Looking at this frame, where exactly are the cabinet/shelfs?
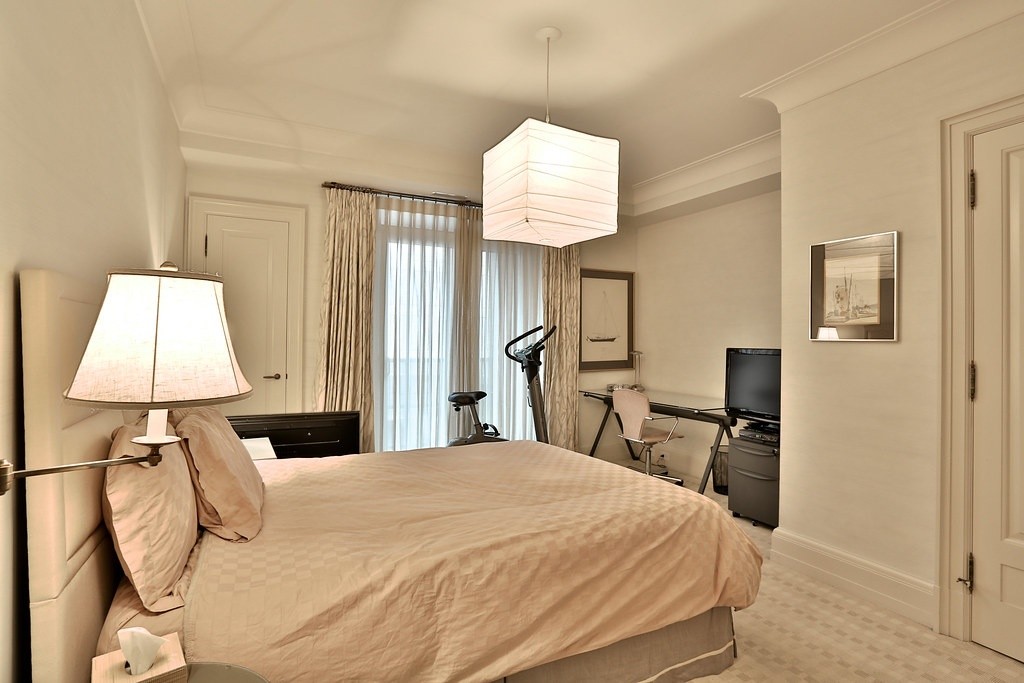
[729,438,780,530]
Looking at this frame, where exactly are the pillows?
[102,401,267,615]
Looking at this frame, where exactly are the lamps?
[1,262,254,495]
[483,26,620,249]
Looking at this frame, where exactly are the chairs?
[612,388,684,486]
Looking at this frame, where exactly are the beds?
[96,441,762,683]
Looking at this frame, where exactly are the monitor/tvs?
[725,348,780,434]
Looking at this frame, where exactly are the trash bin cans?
[710,444,730,496]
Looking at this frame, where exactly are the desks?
[579,387,738,495]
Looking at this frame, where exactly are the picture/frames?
[809,230,900,342]
[579,268,635,373]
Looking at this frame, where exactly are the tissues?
[91,627,188,683]
